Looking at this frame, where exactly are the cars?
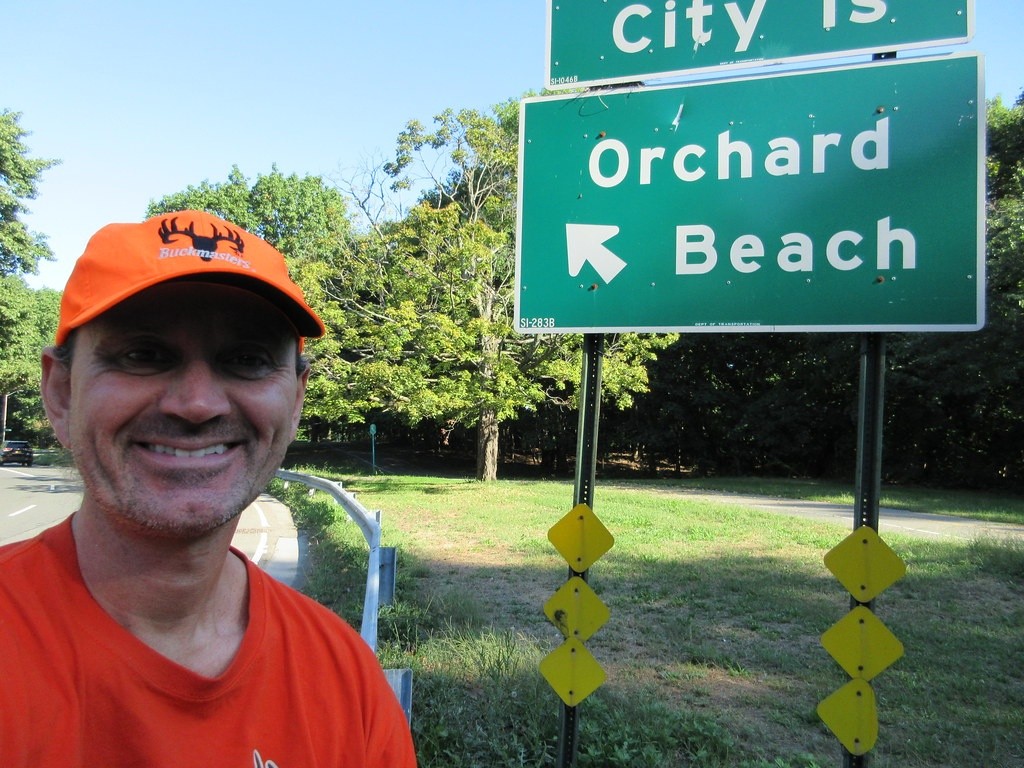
[0,441,33,467]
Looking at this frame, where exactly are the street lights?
[2,390,24,441]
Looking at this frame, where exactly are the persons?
[0,209,417,767]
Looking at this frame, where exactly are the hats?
[55,209,324,354]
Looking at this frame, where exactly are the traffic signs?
[512,0,987,335]
